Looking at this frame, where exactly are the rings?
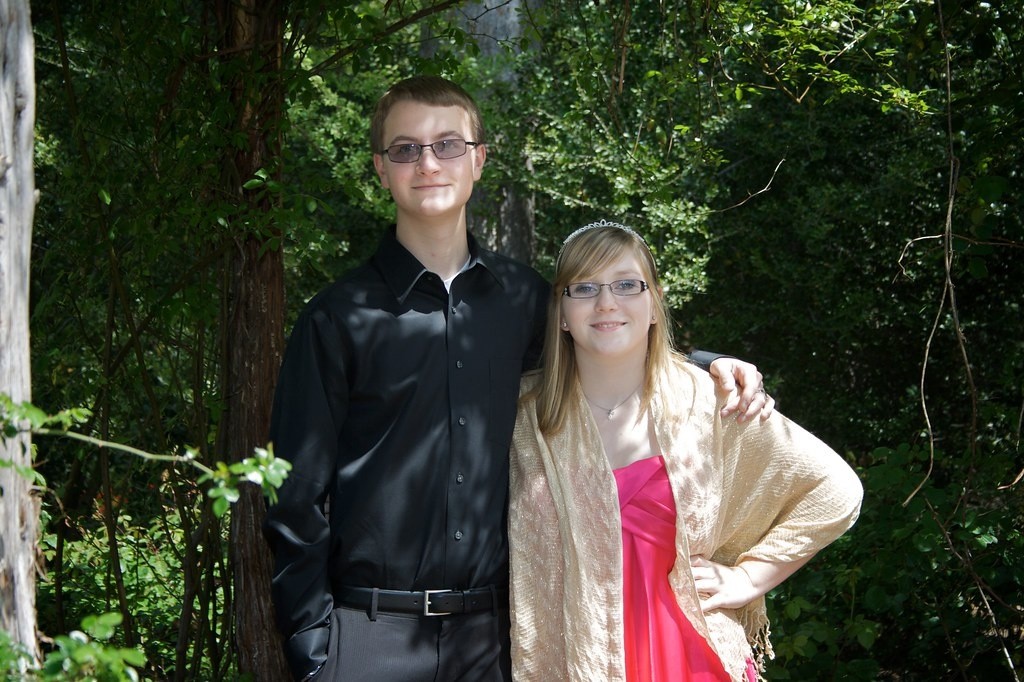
[756,390,768,400]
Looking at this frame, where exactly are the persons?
[261,76,775,682]
[507,218,863,682]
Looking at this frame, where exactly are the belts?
[338,583,509,617]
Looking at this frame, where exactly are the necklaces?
[584,377,644,421]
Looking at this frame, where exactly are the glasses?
[562,279,649,299]
[381,139,478,163]
